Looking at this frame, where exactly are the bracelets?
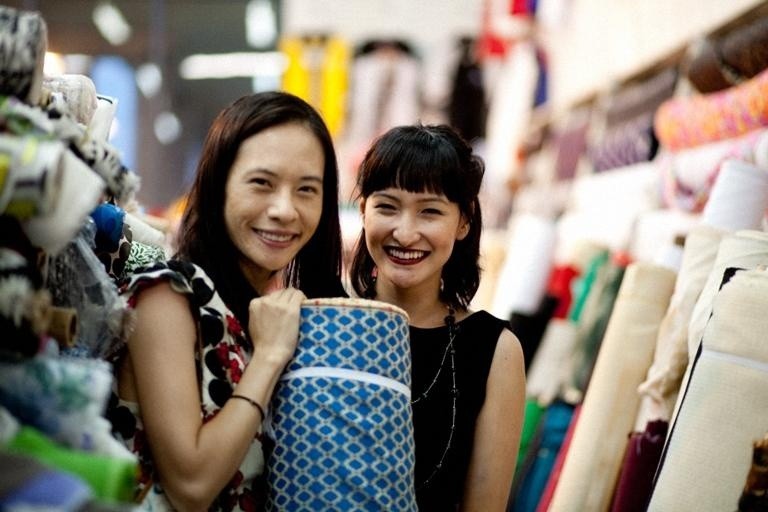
[223,390,273,424]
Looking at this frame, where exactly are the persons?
[344,121,529,512]
[94,85,348,509]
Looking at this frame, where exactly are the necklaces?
[402,303,466,495]
